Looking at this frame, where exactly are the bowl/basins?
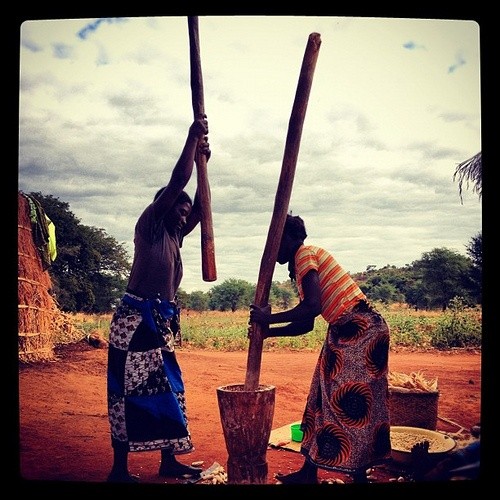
[290,423,306,442]
[387,425,457,464]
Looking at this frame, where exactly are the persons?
[247,213,391,493]
[105,112,212,485]
[409,441,481,494]
